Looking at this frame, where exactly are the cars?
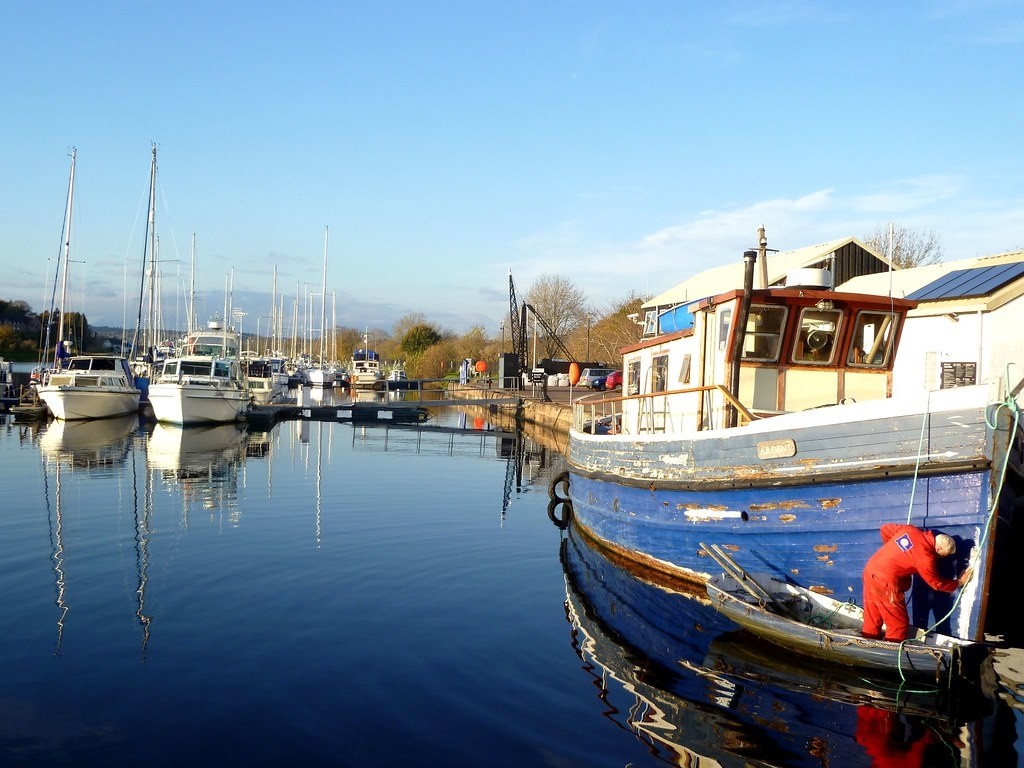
[591,375,607,392]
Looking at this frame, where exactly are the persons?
[859,523,971,644]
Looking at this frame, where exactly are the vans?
[579,367,615,390]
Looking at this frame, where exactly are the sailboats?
[1,143,407,423]
[21,423,337,662]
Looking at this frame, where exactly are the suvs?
[605,369,623,394]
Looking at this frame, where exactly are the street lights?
[500,325,505,352]
[586,312,591,366]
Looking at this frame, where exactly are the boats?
[549,502,1024,768]
[548,219,1023,687]
